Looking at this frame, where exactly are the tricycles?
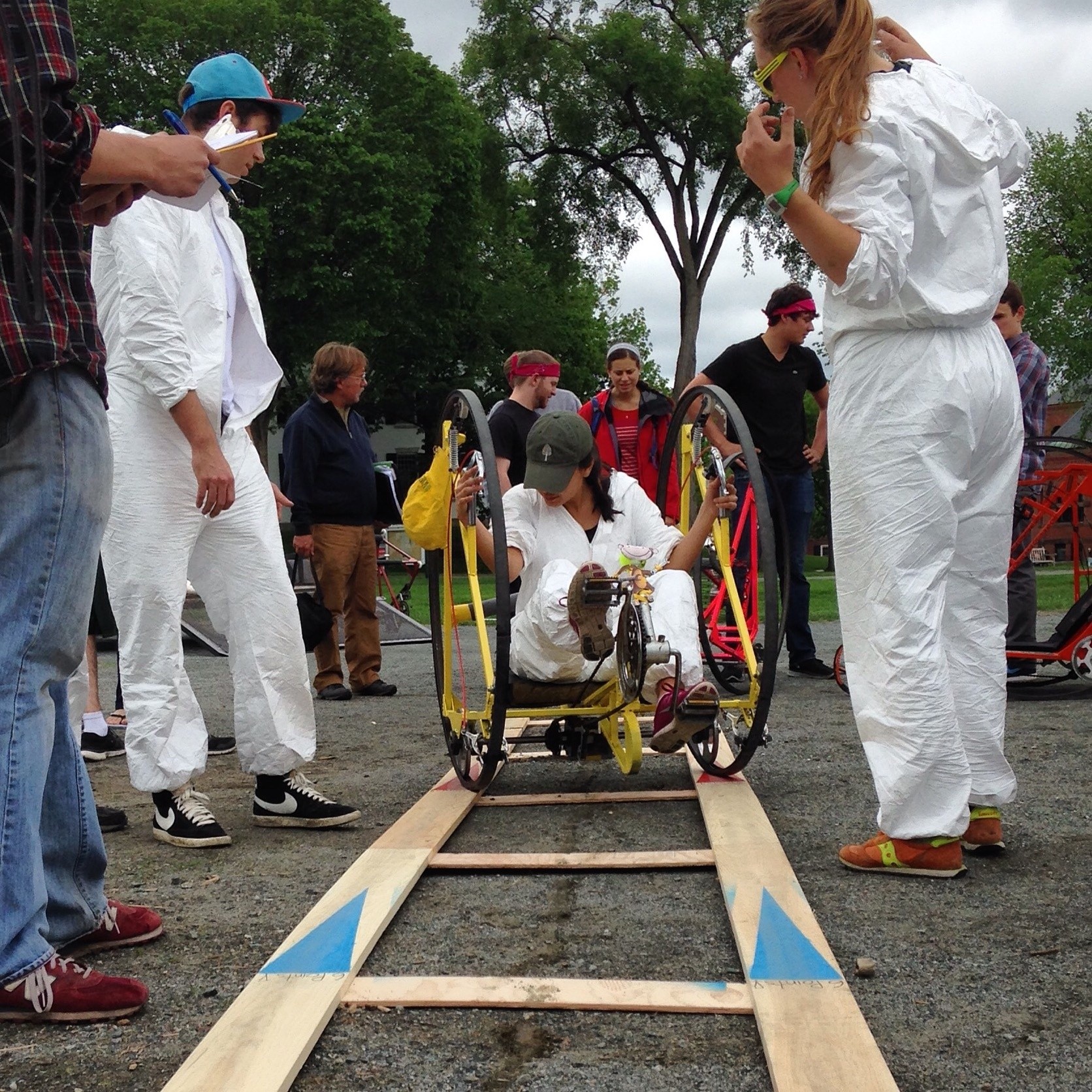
[834,432,1092,695]
[429,386,781,793]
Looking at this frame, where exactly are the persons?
[680,285,829,679]
[577,343,681,526]
[78,612,234,761]
[90,54,365,847]
[283,341,400,696]
[489,389,582,417]
[993,279,1050,674]
[455,409,737,754]
[736,0,1033,877]
[486,350,560,613]
[0,0,220,1025]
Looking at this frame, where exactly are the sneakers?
[1007,666,1037,680]
[0,956,149,1020]
[559,561,615,662]
[252,772,361,827]
[80,726,126,760]
[650,682,719,754]
[206,734,236,754]
[961,803,1006,853]
[95,804,128,831]
[722,667,745,682]
[839,830,966,878]
[56,899,161,960]
[787,657,834,679]
[355,679,397,696]
[153,789,232,847]
[317,683,351,700]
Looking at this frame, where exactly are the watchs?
[764,180,798,214]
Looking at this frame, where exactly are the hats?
[183,53,306,126]
[402,447,452,551]
[523,410,592,494]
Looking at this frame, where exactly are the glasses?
[344,373,367,383]
[754,49,788,96]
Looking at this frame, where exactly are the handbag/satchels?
[372,461,406,524]
[295,591,333,653]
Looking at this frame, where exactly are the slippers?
[109,712,126,726]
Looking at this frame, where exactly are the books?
[373,458,401,525]
